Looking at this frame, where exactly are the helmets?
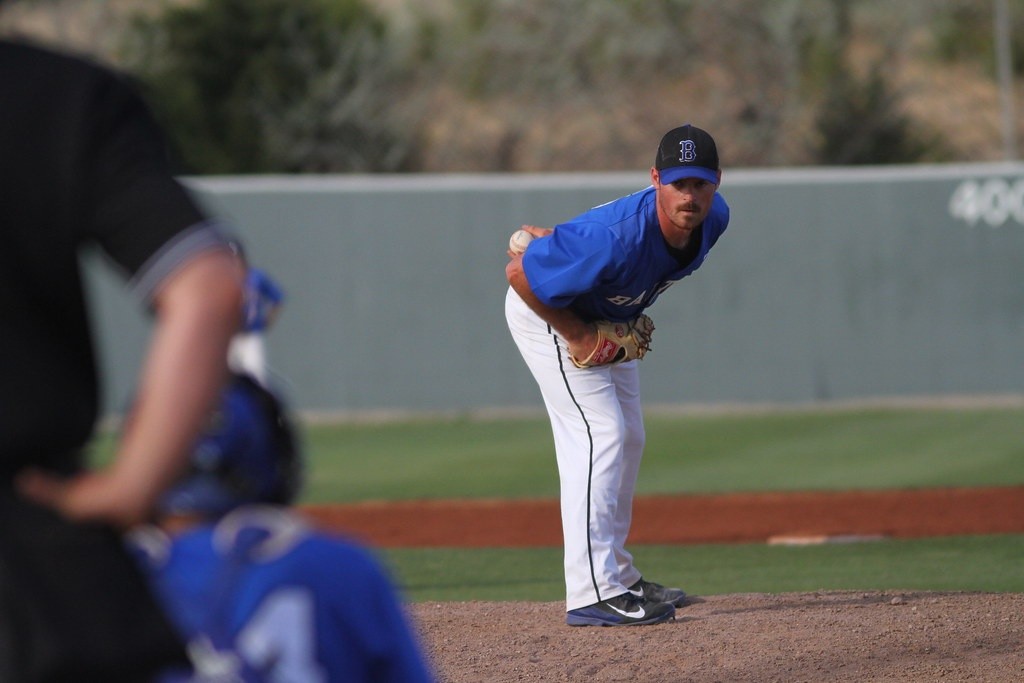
[155,369,303,520]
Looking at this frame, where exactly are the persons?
[0,38,247,683]
[226,237,284,382]
[125,375,435,683]
[504,123,729,626]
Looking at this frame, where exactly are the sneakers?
[566,591,675,626]
[627,578,685,604]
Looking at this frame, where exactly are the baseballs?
[510,229,534,254]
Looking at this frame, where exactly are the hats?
[656,122,720,186]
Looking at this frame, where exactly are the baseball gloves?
[566,313,655,369]
